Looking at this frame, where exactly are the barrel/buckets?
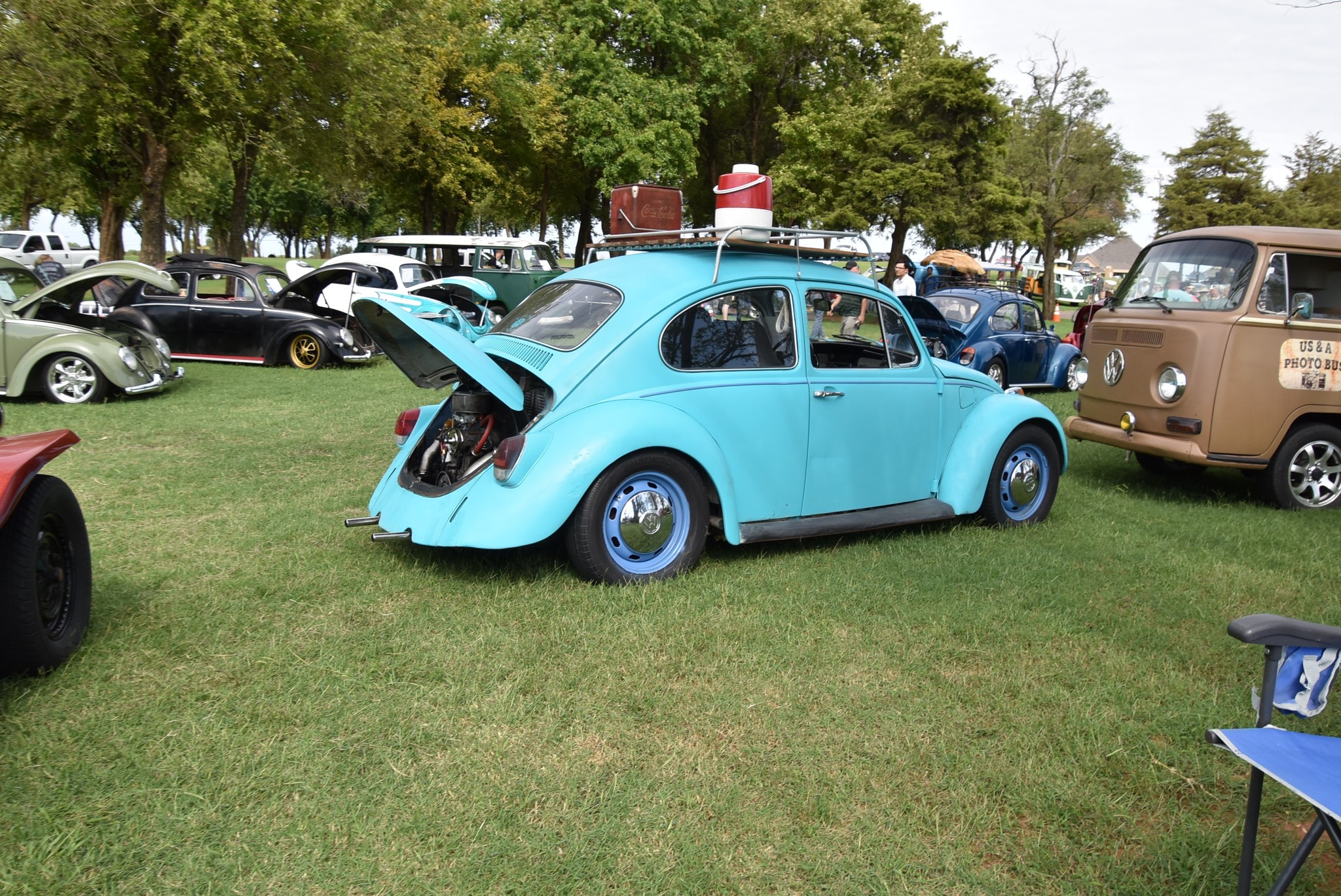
[712,164,774,243]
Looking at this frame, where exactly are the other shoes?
[819,336,829,342]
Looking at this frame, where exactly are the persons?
[1091,272,1105,303]
[892,260,917,298]
[488,249,506,269]
[808,260,833,341]
[33,254,67,293]
[995,271,1009,291]
[961,271,978,289]
[919,267,939,296]
[1129,269,1232,303]
[826,261,868,349]
[720,295,732,321]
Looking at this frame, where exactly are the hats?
[1139,278,1151,283]
[1092,275,1097,279]
[843,261,858,269]
[1096,272,1101,275]
[1196,286,1211,293]
[1166,271,1182,280]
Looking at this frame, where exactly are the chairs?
[711,319,783,367]
[675,308,713,329]
[227,297,247,301]
[1197,612,1341,896]
[207,297,226,300]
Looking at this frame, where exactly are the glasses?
[894,266,907,271]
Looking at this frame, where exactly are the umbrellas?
[919,249,986,287]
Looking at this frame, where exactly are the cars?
[0,230,187,403]
[1018,264,1091,303]
[346,228,1066,584]
[107,253,386,370]
[286,228,569,342]
[1062,225,1341,511]
[876,276,1082,393]
[1,405,93,678]
